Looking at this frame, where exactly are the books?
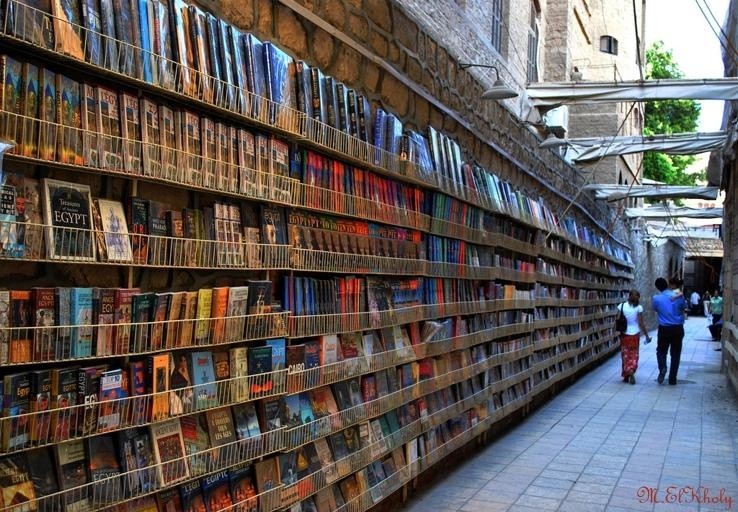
[1,1,633,512]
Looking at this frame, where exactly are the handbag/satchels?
[616,311,627,332]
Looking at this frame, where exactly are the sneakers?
[630,377,635,385]
[623,378,629,383]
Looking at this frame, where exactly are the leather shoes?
[658,368,667,384]
[669,380,677,385]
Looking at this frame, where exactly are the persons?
[667,277,683,301]
[615,289,649,386]
[651,277,683,385]
[685,285,723,342]
